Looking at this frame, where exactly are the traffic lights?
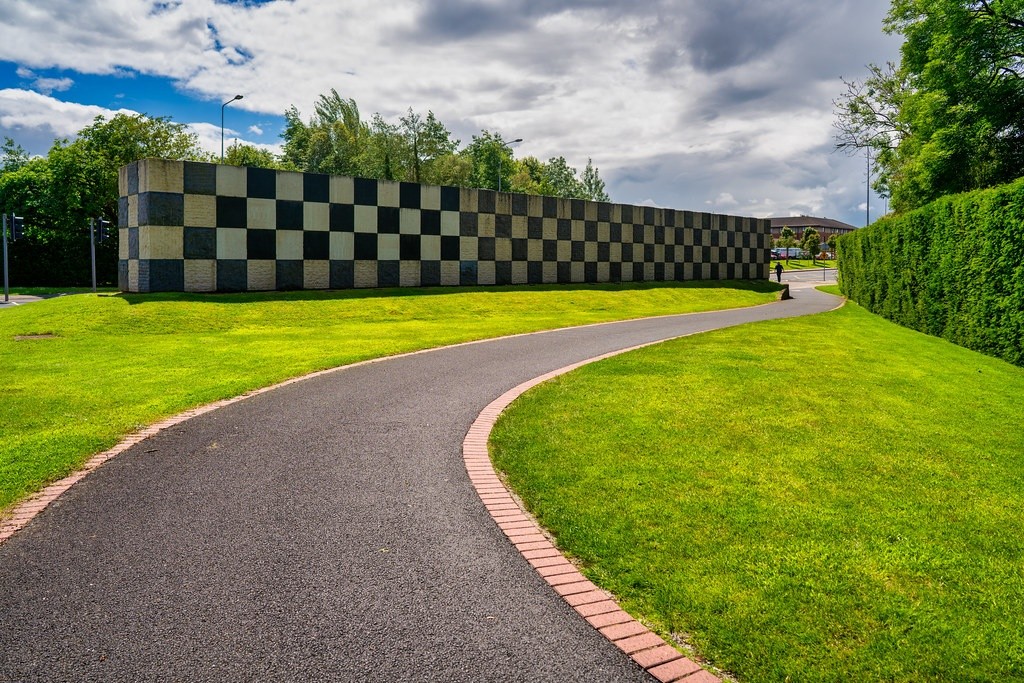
[97,216,109,244]
[11,212,25,242]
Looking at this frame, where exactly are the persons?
[775,262,784,282]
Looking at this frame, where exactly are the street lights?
[498,138,523,191]
[836,143,870,227]
[221,94,254,164]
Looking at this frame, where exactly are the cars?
[769,247,835,261]
